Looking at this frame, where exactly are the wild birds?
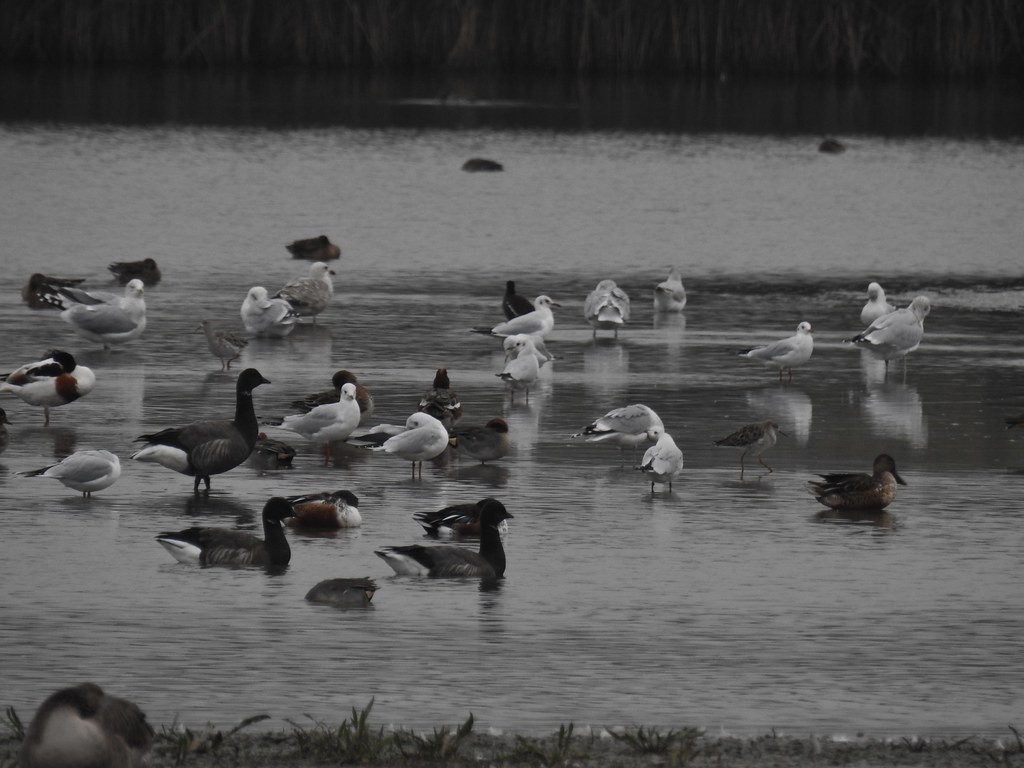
[16,450,122,500]
[9,682,160,768]
[0,348,97,427]
[155,496,303,571]
[19,258,162,311]
[193,137,932,613]
[130,368,272,502]
[59,279,148,356]
